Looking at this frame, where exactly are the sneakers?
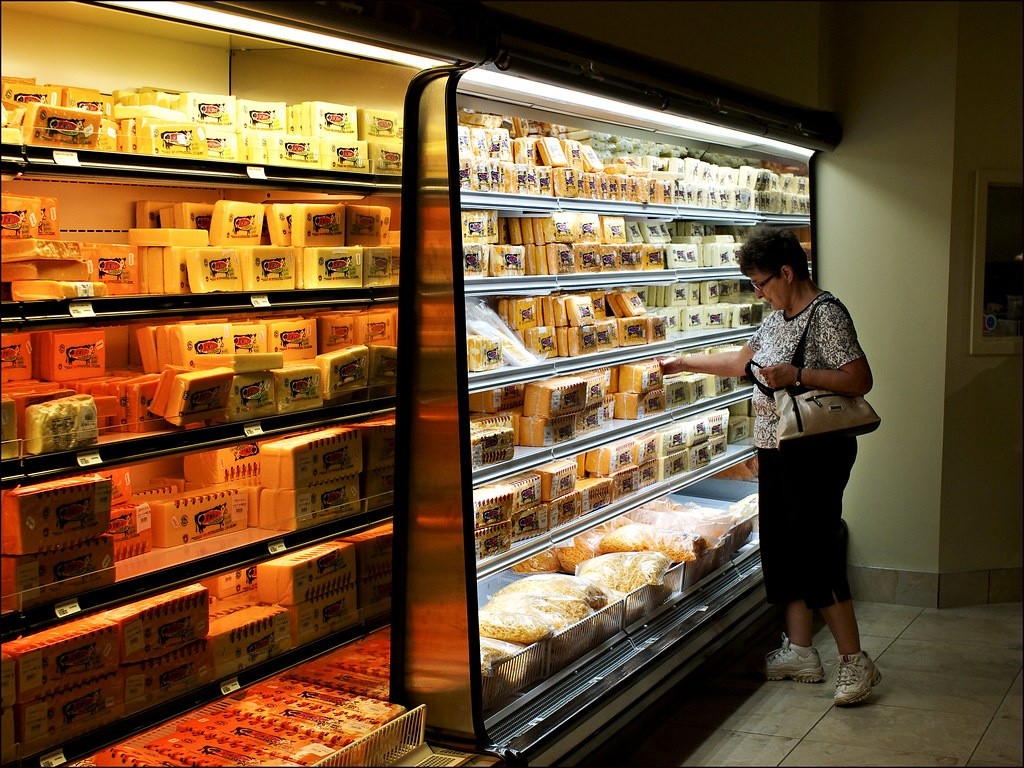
[753,631,824,683]
[824,650,881,704]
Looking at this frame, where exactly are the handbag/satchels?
[745,299,880,449]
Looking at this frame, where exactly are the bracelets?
[796,367,802,386]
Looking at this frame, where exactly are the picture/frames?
[969,170,1024,355]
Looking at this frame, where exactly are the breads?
[0,72,401,767]
[458,108,762,169]
[455,127,809,557]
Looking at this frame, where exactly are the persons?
[658,227,881,705]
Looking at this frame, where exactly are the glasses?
[750,271,779,291]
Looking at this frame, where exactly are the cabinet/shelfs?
[459,20,844,734]
[0,0,457,768]
